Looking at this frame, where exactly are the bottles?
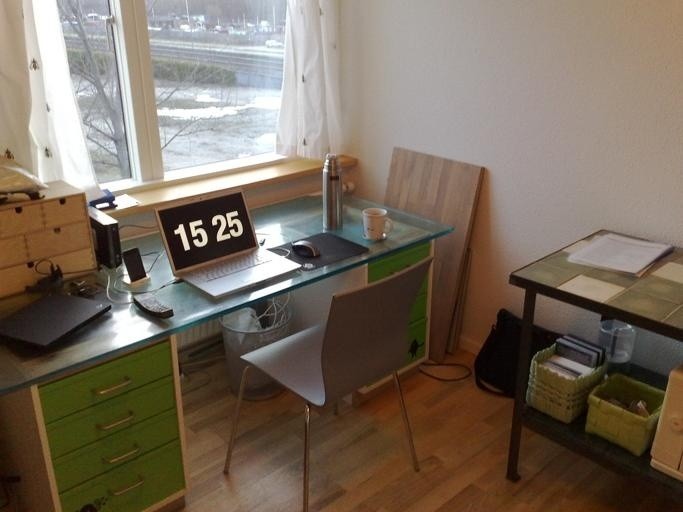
[321,152,344,230]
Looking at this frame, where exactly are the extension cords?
[33,275,63,292]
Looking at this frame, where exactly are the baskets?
[525,342,609,424]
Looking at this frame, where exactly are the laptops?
[0,291,115,349]
[155,187,302,299]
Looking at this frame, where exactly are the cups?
[360,207,393,241]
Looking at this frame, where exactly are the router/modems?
[87,206,122,268]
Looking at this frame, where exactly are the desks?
[0,190,453,511]
[506,228,682,502]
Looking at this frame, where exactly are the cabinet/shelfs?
[1,180,97,299]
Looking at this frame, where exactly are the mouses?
[293,240,320,257]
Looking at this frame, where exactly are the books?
[566,232,674,278]
[537,334,605,380]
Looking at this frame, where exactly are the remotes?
[133,292,174,319]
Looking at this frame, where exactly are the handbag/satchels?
[474,309,564,401]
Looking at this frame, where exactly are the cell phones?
[123,248,146,282]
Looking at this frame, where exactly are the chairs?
[220,254,433,512]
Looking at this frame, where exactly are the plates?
[361,232,386,240]
